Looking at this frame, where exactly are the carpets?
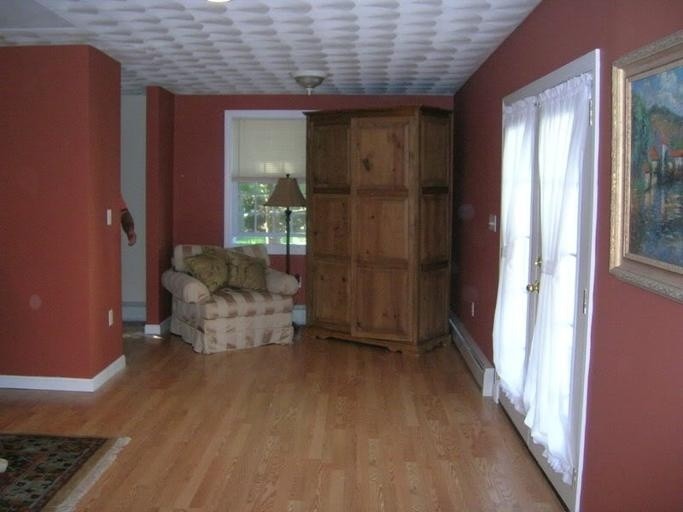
[0,433,133,511]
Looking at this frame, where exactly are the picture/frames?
[608,28,682,305]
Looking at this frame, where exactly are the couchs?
[161,241,300,358]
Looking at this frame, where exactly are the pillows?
[226,247,266,292]
[186,253,225,293]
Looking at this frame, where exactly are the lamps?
[264,174,305,275]
[288,68,327,96]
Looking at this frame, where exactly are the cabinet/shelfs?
[301,105,456,358]
